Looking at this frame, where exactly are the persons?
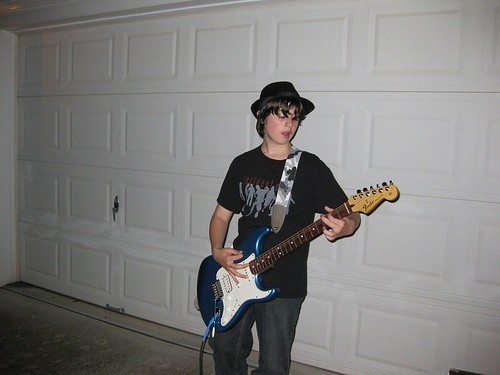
[209,81,361,375]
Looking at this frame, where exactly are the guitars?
[195,180,399,332]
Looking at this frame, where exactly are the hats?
[250,81,315,119]
